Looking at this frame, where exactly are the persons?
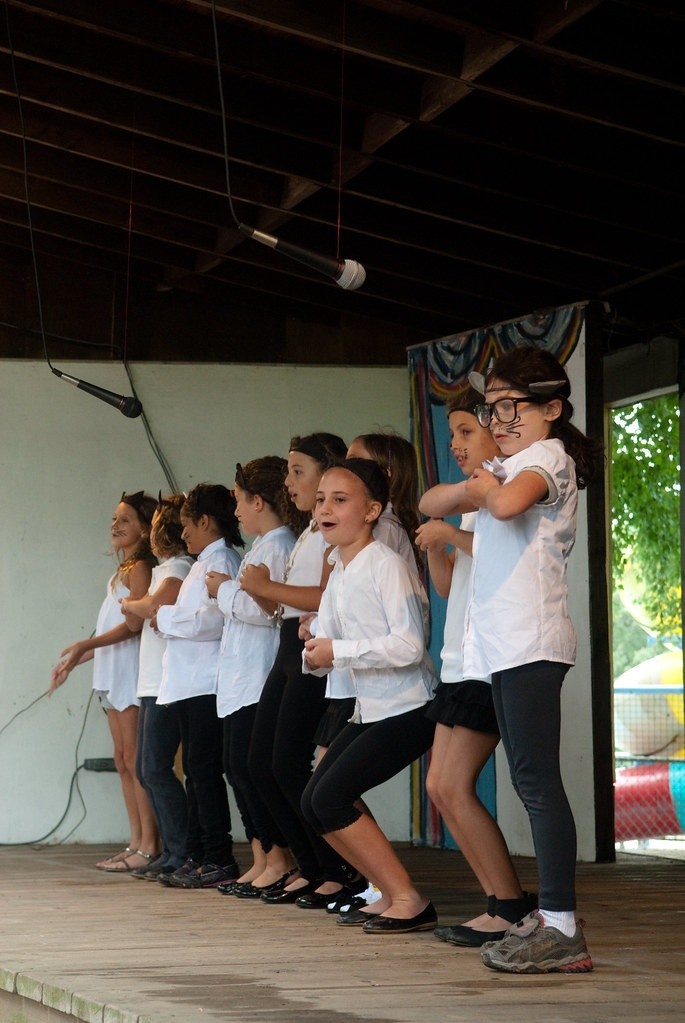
[261,432,348,908]
[418,345,608,974]
[204,456,311,898]
[149,483,246,888]
[414,391,538,947]
[47,488,197,881]
[300,457,439,933]
[298,433,426,916]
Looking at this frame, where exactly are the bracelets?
[262,601,284,621]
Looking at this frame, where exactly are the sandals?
[104,849,165,872]
[96,845,137,870]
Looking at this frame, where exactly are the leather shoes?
[259,874,321,904]
[233,865,301,897]
[218,873,250,894]
[295,876,369,908]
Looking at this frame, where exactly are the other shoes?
[362,901,438,932]
[433,910,496,942]
[336,908,378,927]
[170,862,242,888]
[447,919,508,948]
[157,858,203,886]
[130,854,162,878]
[143,855,181,881]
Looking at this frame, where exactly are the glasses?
[474,395,535,428]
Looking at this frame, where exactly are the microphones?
[236,222,366,292]
[51,369,142,419]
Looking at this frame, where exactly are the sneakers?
[480,909,593,973]
